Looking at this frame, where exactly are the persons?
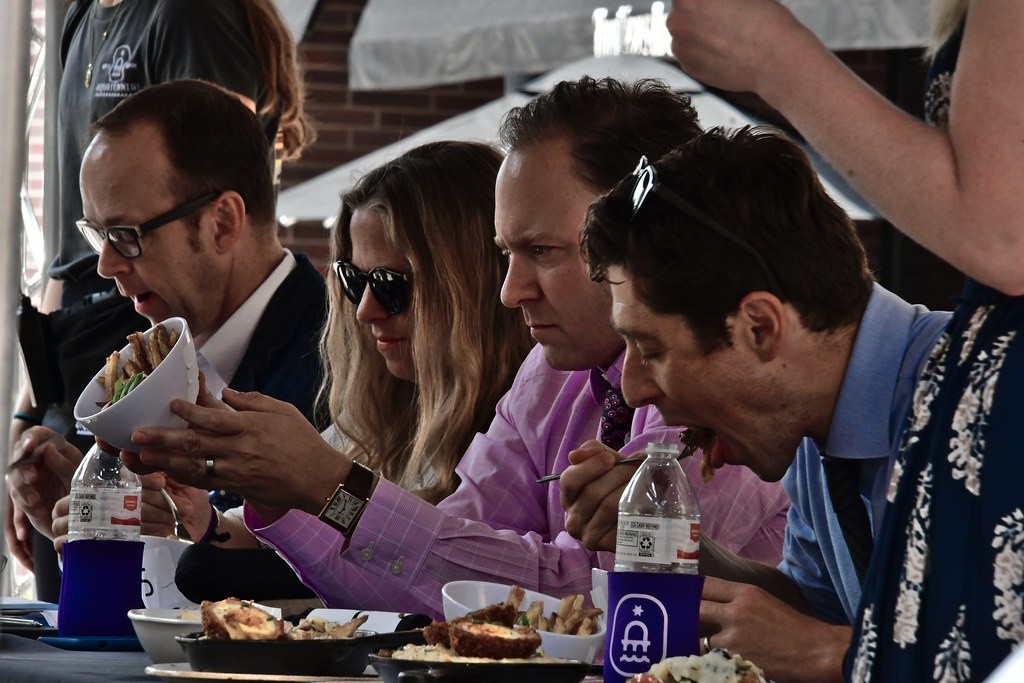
[666,1,1024,683]
[7,1,334,592]
[556,126,955,683]
[51,140,540,589]
[94,69,791,626]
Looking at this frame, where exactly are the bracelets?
[203,505,221,543]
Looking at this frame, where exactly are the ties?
[600,384,635,451]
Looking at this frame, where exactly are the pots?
[366,651,603,682]
[174,631,425,675]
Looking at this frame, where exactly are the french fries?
[97,324,179,411]
[505,586,603,635]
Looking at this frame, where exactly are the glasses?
[627,154,786,302]
[75,191,248,257]
[332,257,413,316]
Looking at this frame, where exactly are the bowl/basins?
[592,569,608,627]
[443,579,607,664]
[128,600,281,661]
[57,533,194,610]
[72,316,199,451]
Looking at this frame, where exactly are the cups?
[603,572,705,683]
[58,540,147,636]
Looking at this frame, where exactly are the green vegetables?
[112,371,146,404]
[396,601,542,657]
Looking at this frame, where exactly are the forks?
[536,430,705,486]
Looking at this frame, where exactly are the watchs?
[317,460,374,536]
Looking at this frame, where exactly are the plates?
[146,662,381,683]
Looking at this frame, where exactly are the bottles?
[69,440,142,541]
[614,441,700,575]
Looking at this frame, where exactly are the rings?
[204,458,214,477]
[703,635,711,653]
